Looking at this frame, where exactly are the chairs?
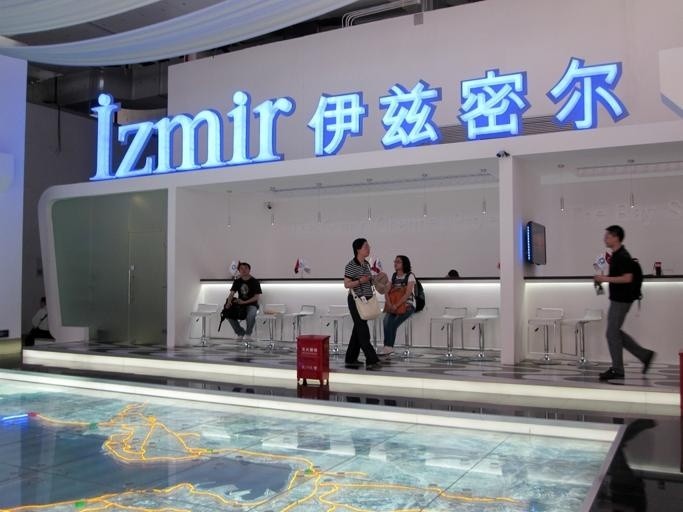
[256,302,287,352]
[463,307,500,361]
[431,305,468,361]
[371,306,424,359]
[235,303,262,349]
[283,304,316,353]
[319,304,350,356]
[190,302,219,348]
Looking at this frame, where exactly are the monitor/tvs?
[525,220,547,265]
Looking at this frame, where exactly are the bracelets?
[358,278,361,285]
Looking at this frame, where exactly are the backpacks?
[405,272,425,313]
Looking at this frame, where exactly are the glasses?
[393,260,403,264]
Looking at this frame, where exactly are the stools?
[527,308,603,366]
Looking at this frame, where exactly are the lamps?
[227,191,231,227]
[268,168,492,227]
[627,160,636,208]
[557,164,566,211]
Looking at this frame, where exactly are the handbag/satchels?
[630,257,642,299]
[384,287,407,313]
[223,298,248,320]
[354,295,381,320]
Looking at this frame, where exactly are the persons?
[25,295,52,346]
[225,264,263,342]
[592,225,655,380]
[343,238,388,368]
[376,255,416,358]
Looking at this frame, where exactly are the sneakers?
[236,334,252,342]
[599,367,623,379]
[641,350,653,374]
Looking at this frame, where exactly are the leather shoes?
[345,345,393,366]
